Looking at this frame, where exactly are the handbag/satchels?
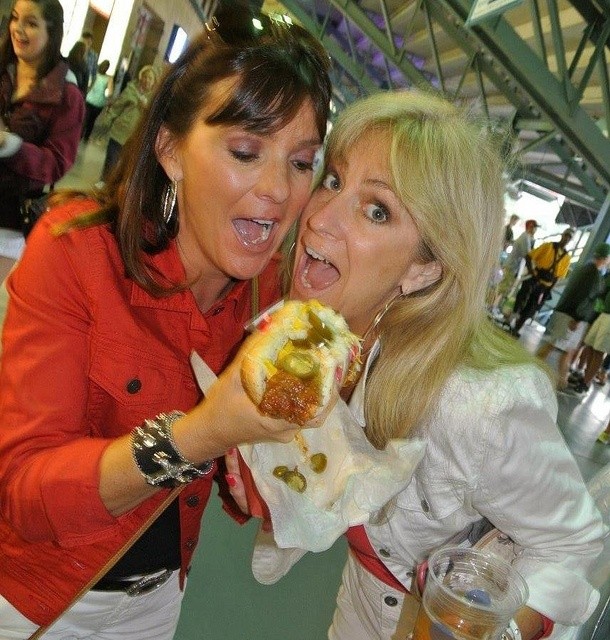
[16,183,54,243]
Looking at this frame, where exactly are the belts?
[90,570,176,599]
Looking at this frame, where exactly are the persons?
[80,57,110,144]
[493,220,541,315]
[287,83,609,639]
[65,42,89,96]
[0,0,88,233]
[534,241,610,399]
[505,227,576,340]
[80,31,98,77]
[1,19,335,636]
[502,214,523,250]
[568,300,610,394]
[93,60,160,188]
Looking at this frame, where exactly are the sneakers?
[596,432,609,444]
[556,383,576,398]
[567,372,589,396]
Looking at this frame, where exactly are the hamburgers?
[241,301,366,429]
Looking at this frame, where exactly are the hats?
[593,242,610,257]
[563,229,574,238]
[525,220,541,229]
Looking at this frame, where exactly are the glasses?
[185,7,331,74]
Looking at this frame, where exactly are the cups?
[412,546,530,640]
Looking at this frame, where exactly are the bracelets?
[128,408,217,493]
